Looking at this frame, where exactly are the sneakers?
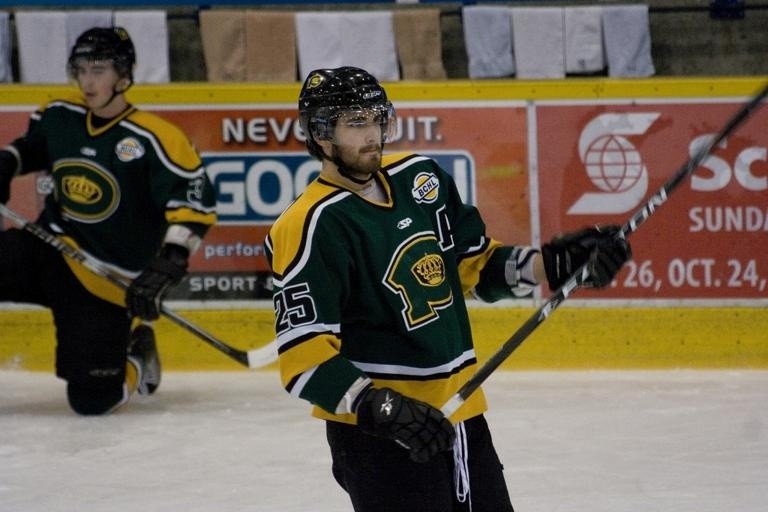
[132,324,160,396]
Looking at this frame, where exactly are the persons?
[263,67,632,512]
[0,27,217,414]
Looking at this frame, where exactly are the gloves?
[540,226,632,293]
[124,259,186,322]
[358,386,456,464]
[1,150,18,206]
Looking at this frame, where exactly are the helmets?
[298,66,388,139]
[68,27,136,65]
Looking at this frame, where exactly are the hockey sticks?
[0,205,278,370]
